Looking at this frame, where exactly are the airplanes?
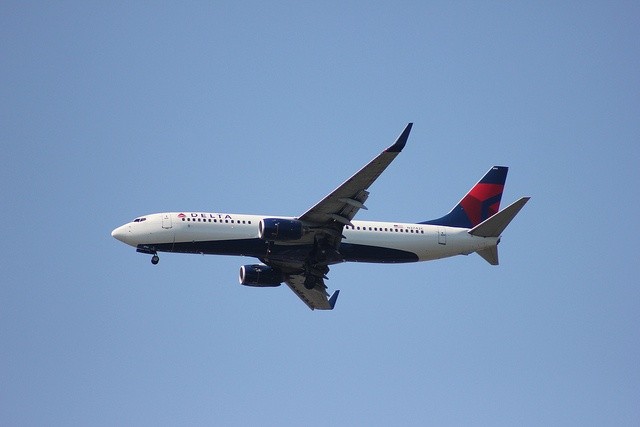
[110,122,530,311]
